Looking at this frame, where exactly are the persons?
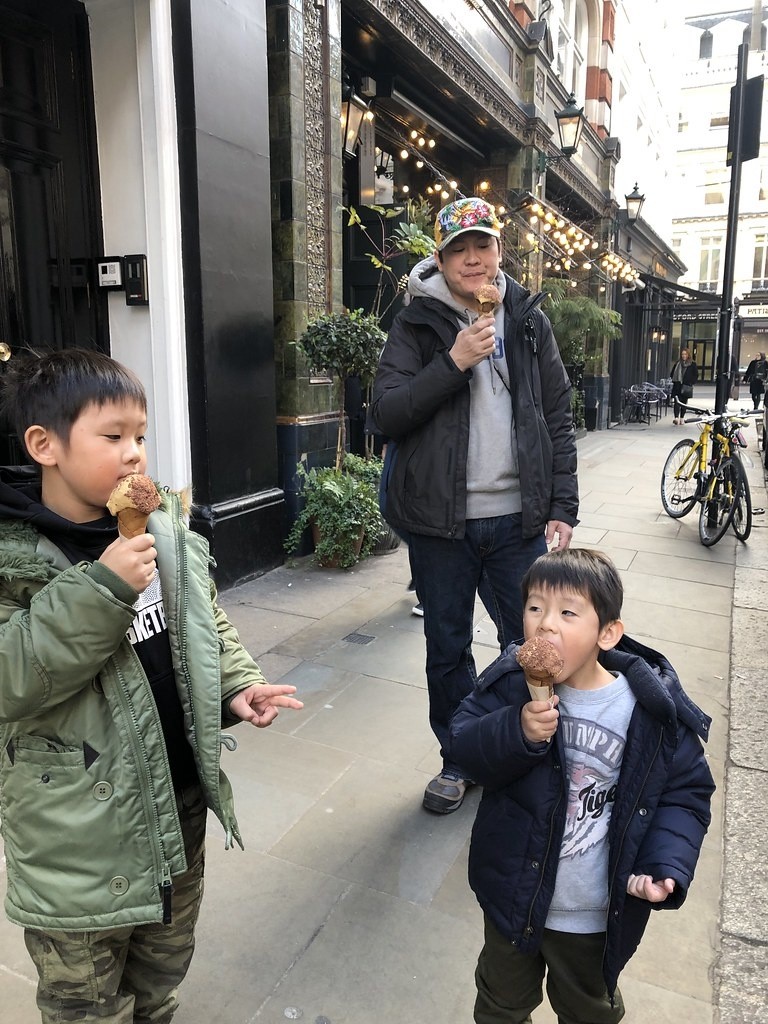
[0,353,307,1024]
[742,351,768,410]
[371,198,587,817]
[667,349,699,425]
[714,346,738,405]
[450,545,713,1024]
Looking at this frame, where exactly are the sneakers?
[423,771,476,815]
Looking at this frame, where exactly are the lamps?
[659,329,667,344]
[653,325,661,343]
[554,91,585,155]
[341,81,369,167]
[625,182,646,222]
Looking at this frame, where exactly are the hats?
[434,196,500,250]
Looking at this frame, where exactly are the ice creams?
[473,284,502,316]
[106,473,163,542]
[515,636,565,743]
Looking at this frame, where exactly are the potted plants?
[285,466,372,568]
[342,453,401,556]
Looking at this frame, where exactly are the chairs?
[631,385,657,425]
[642,381,667,419]
[658,378,674,408]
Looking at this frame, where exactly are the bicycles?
[673,408,765,541]
[660,396,751,548]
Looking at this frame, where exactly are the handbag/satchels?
[680,385,694,398]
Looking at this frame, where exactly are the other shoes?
[673,419,684,425]
